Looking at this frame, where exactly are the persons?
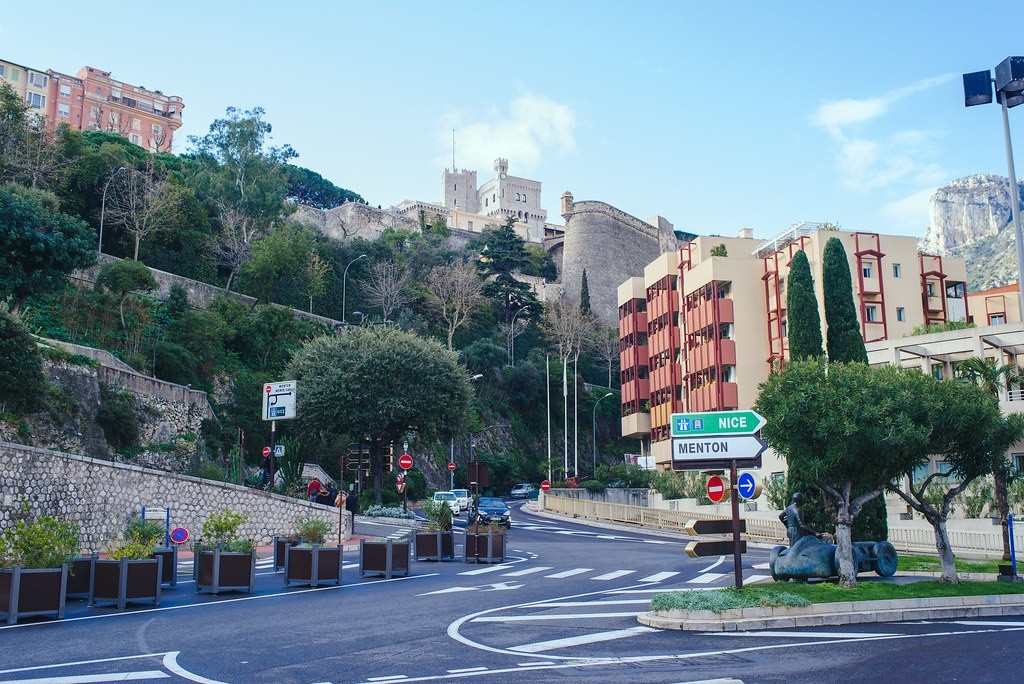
[777,492,822,547]
[334,490,347,510]
[346,492,358,516]
[307,477,321,502]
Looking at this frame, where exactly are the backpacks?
[311,483,318,496]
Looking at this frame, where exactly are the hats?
[313,477,317,480]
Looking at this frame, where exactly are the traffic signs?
[382,440,393,472]
[346,443,371,471]
[671,435,769,460]
[671,409,767,438]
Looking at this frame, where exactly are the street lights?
[451,373,483,489]
[593,392,613,480]
[98,167,126,254]
[353,311,364,329]
[343,255,368,322]
[512,305,532,368]
[470,424,511,461]
[962,55,1024,306]
[403,441,408,513]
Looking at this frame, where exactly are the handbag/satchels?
[336,495,346,506]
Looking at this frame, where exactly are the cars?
[509,484,535,498]
[449,489,474,511]
[467,497,512,529]
[432,491,461,516]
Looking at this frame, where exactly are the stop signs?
[707,476,730,503]
[448,462,455,470]
[541,480,550,491]
[266,386,271,392]
[399,455,414,470]
[262,446,271,457]
